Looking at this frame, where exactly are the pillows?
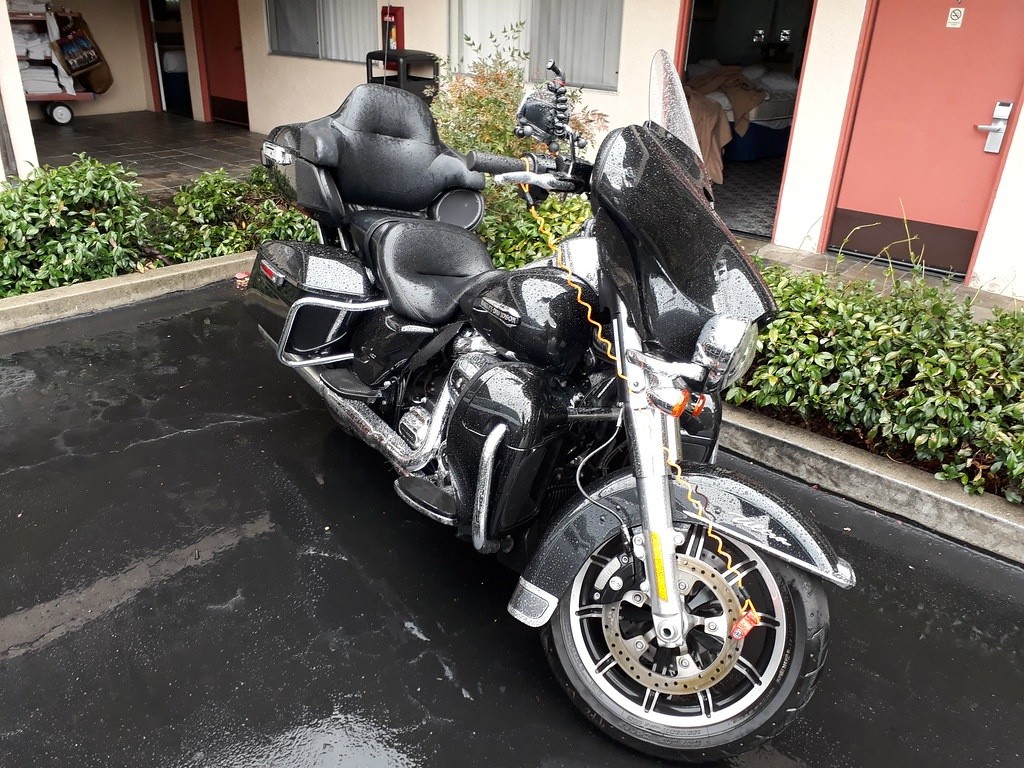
[761,71,798,91]
[742,63,769,80]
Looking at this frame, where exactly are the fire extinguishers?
[389,25,396,50]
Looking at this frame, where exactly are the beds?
[682,60,799,129]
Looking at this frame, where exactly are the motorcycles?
[239,48,861,768]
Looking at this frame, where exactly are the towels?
[6,0,77,96]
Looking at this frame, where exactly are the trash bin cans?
[366,49,440,107]
